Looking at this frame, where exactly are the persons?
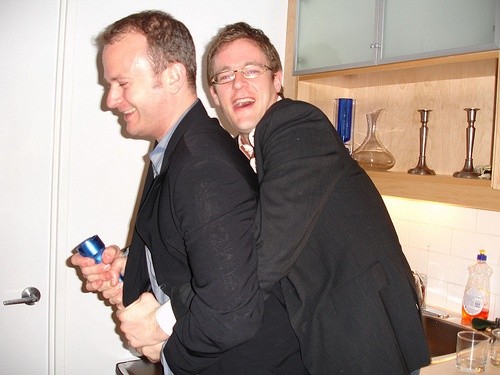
[71,9,307,375]
[97,22,432,375]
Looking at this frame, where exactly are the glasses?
[209,64,274,86]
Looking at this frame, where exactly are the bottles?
[350,108,395,170]
[462,250,492,325]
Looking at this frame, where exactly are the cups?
[490,328,500,365]
[456,331,490,373]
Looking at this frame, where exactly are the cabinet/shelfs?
[283,0,500,357]
